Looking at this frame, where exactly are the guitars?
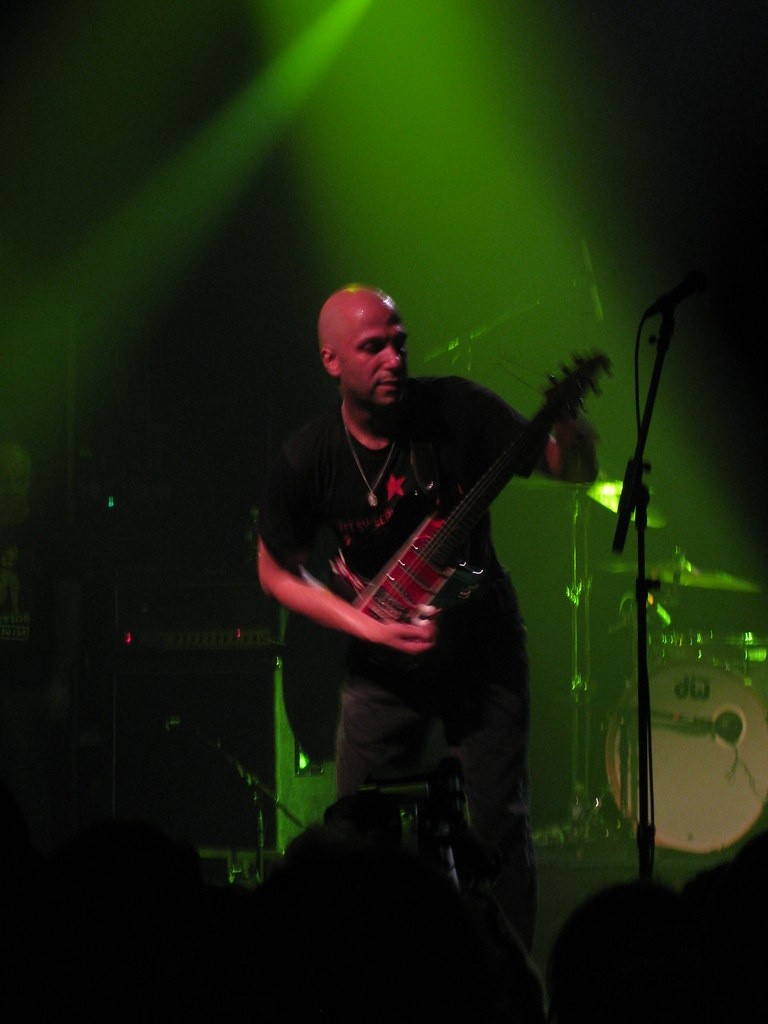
[322,345,616,672]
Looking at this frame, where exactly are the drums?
[597,651,768,856]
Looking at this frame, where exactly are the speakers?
[111,657,277,886]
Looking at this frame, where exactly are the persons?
[256,282,599,951]
[0,441,84,853]
[0,782,768,1024]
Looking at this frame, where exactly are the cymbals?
[585,479,671,529]
[589,546,764,596]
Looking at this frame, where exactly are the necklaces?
[345,411,397,506]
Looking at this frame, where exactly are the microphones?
[643,269,702,322]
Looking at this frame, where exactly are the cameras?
[355,776,471,856]
